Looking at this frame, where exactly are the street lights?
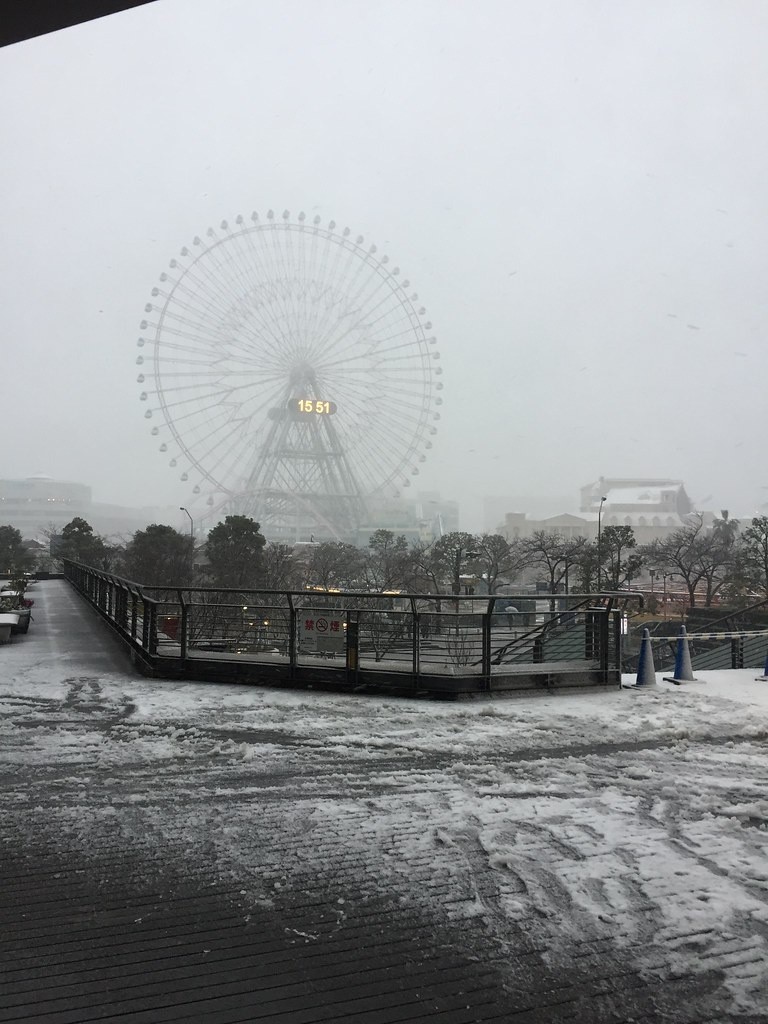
[455,552,482,637]
[663,575,666,621]
[181,507,193,539]
[650,569,654,595]
[598,497,607,591]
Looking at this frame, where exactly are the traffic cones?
[662,624,705,686]
[623,627,657,691]
